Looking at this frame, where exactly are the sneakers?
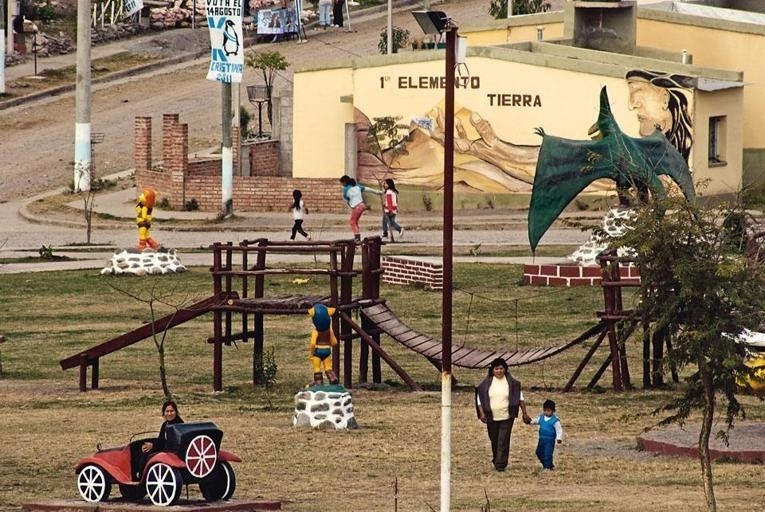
[399,228,404,237]
[381,236,387,241]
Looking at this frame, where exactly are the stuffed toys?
[308,302,339,385]
[134,188,162,252]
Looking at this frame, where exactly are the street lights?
[413,3,468,511]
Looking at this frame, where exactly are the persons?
[381,177,403,239]
[474,357,531,473]
[135,402,185,478]
[526,399,562,470]
[331,0,345,28]
[317,0,332,29]
[288,190,312,239]
[427,69,693,199]
[269,13,281,29]
[338,174,386,243]
